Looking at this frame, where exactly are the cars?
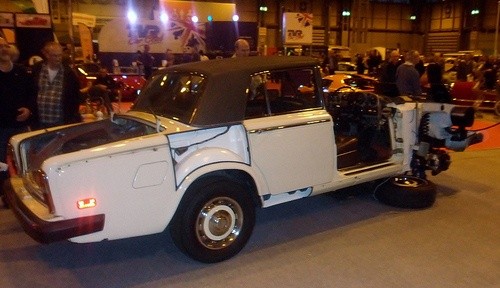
[296,73,380,96]
[321,62,361,74]
[448,78,500,113]
[424,58,467,73]
[7,56,484,263]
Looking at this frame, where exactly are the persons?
[64,34,500,104]
[4,44,34,100]
[92,67,117,119]
[0,37,36,209]
[28,41,82,137]
